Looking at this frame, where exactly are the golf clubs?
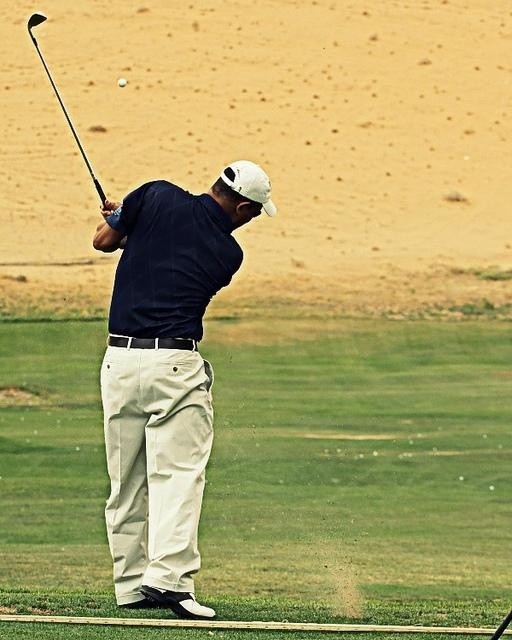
[29,14,106,204]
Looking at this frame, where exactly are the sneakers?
[141,585,215,620]
[118,595,170,610]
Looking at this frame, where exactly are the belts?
[107,331,198,352]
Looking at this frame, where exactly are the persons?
[91,158,277,620]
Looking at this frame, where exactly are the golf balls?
[118,77,127,86]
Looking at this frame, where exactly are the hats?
[220,160,277,219]
